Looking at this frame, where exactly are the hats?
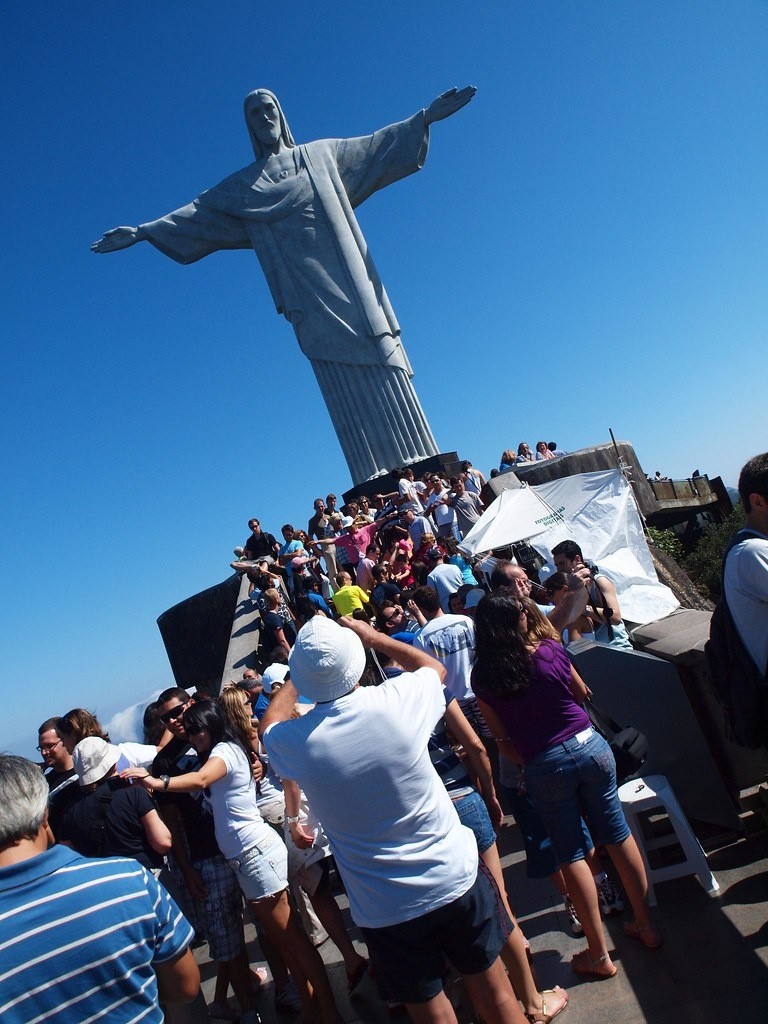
[262,663,291,694]
[399,502,414,512]
[72,737,122,786]
[288,610,367,702]
[463,588,486,609]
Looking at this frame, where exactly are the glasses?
[243,699,250,706]
[431,480,439,483]
[37,739,62,753]
[186,724,204,736]
[400,510,410,517]
[387,609,400,622]
[360,500,366,505]
[160,701,190,725]
[547,588,556,598]
[519,604,525,613]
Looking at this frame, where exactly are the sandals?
[571,949,618,976]
[626,920,660,947]
[524,989,567,1024]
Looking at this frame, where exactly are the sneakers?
[597,876,623,915]
[563,894,582,933]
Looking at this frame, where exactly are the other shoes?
[238,1009,265,1024]
[207,1002,236,1021]
[272,981,302,1011]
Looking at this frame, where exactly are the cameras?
[584,562,599,579]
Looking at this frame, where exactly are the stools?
[617,775,719,908]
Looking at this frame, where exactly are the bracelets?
[285,817,299,824]
[585,605,592,617]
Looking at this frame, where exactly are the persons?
[644,470,665,482]
[90,86,477,486]
[725,451,768,680]
[499,441,567,473]
[0,460,660,1024]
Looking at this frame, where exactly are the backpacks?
[584,700,648,783]
[75,789,115,856]
[691,532,768,746]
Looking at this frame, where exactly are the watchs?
[160,774,170,791]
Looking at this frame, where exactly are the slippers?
[346,957,370,996]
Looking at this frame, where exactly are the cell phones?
[105,774,134,792]
